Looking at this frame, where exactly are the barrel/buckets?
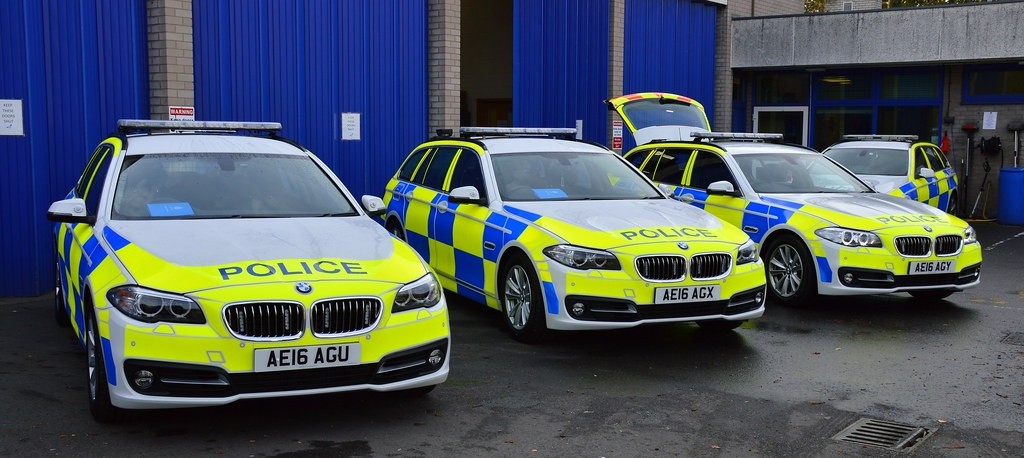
[997,166,1024,224]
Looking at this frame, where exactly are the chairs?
[758,163,803,197]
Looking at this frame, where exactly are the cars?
[382,124,767,342]
[604,90,983,309]
[821,134,959,219]
[49,119,450,421]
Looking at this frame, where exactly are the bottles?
[939,129,949,152]
